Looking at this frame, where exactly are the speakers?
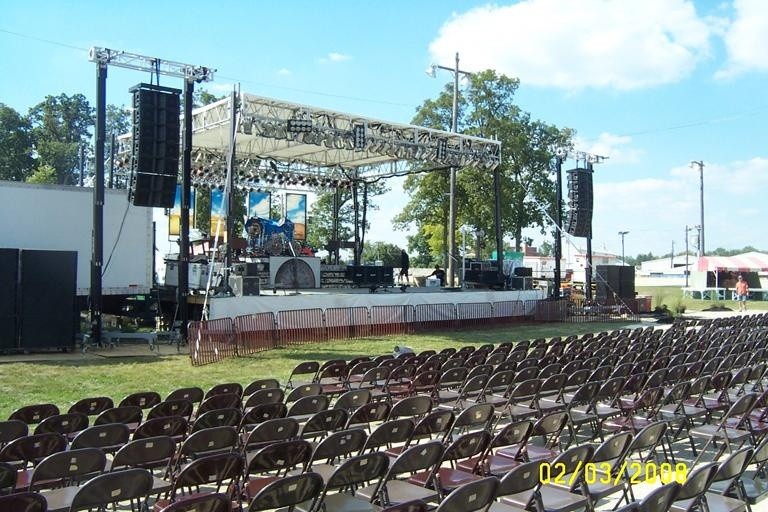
[596,265,618,299]
[19,247,78,352]
[618,265,634,298]
[0,247,19,354]
[565,168,595,237]
[127,91,183,209]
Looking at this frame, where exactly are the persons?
[398,249,409,282]
[424,265,445,286]
[736,275,749,312]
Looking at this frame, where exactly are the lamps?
[236,112,499,172]
